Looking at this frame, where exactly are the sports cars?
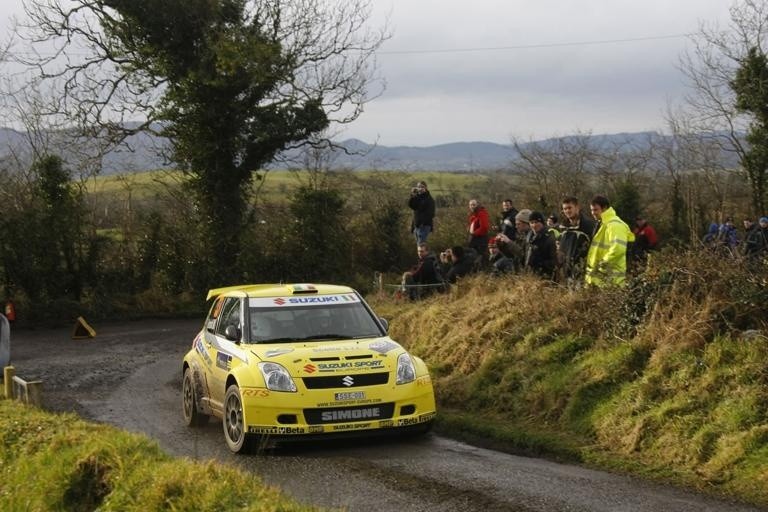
[180,279,436,455]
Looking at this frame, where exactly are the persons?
[702,223,718,242]
[439,192,593,282]
[719,216,738,251]
[630,216,656,253]
[408,179,434,251]
[740,218,763,257]
[400,241,443,299]
[582,196,631,289]
[305,309,333,337]
[758,216,768,252]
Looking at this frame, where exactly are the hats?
[488,237,501,248]
[515,208,531,223]
[529,211,544,225]
[452,246,464,257]
